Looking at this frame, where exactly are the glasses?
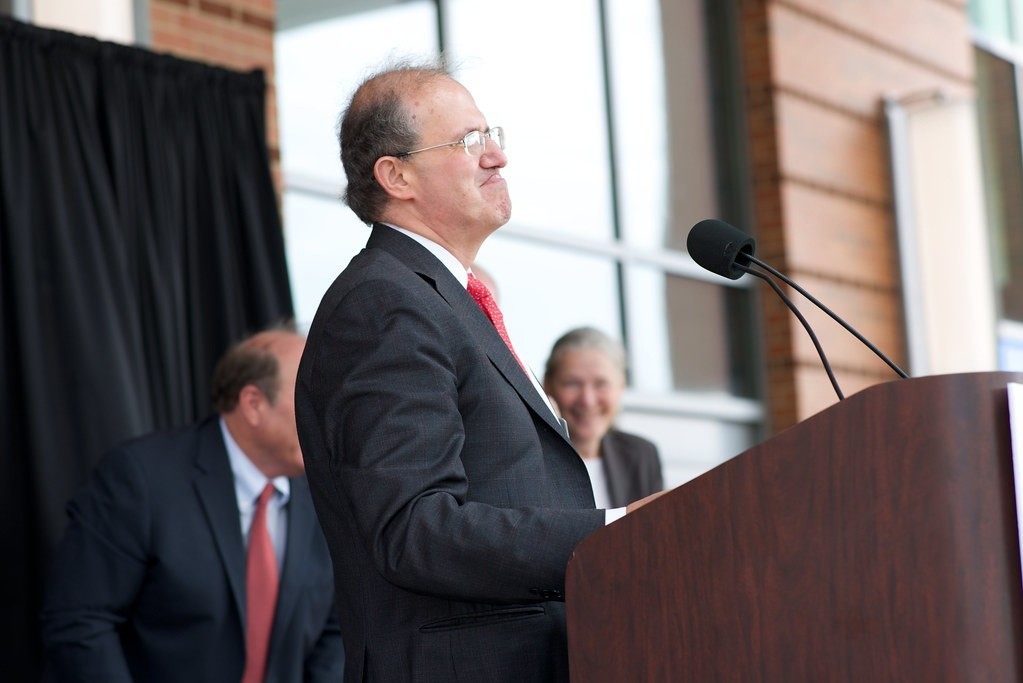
[371,127,505,181]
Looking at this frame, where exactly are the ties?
[466,272,530,380]
[242,483,276,683]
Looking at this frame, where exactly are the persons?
[38,328,350,683]
[295,67,675,683]
[543,327,664,511]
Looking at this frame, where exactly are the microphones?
[686,219,911,399]
